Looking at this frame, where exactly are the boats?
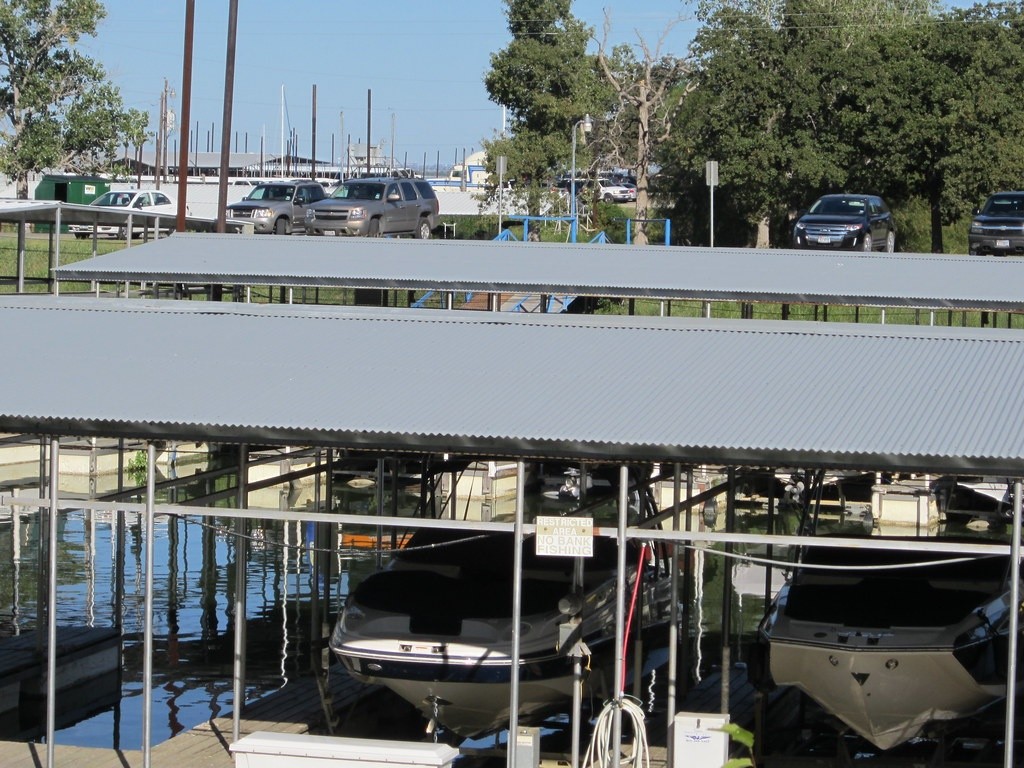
[0,431,340,502]
[758,534,1024,756]
[328,466,687,741]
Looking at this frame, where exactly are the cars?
[509,171,637,206]
[67,189,192,240]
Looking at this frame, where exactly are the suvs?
[793,193,896,253]
[304,176,440,239]
[226,178,331,235]
[968,191,1024,257]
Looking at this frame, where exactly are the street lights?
[571,114,592,243]
[162,80,176,184]
